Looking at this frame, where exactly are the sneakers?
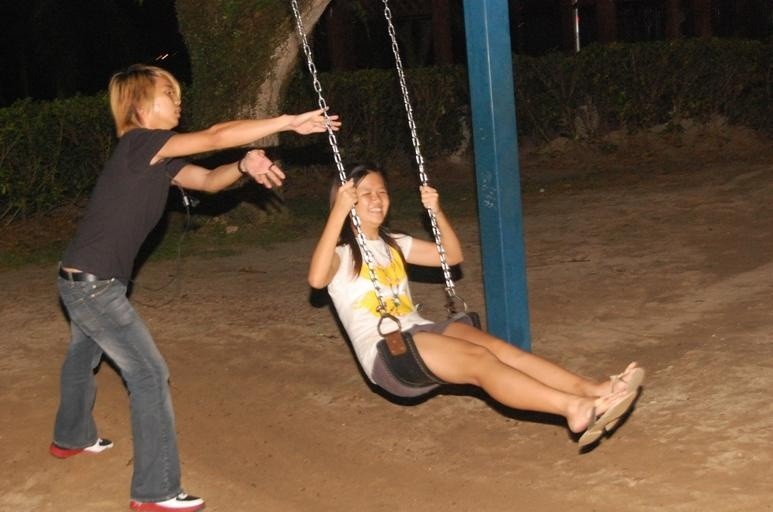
[49,437,113,459]
[131,491,205,512]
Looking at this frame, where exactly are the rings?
[268,163,275,169]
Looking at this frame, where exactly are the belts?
[58,262,116,283]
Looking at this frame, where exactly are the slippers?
[605,367,644,431]
[576,391,637,448]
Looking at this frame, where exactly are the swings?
[290,0,481,387]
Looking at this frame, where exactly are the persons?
[306,160,646,450]
[47,63,343,510]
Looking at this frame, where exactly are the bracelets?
[237,157,248,177]
